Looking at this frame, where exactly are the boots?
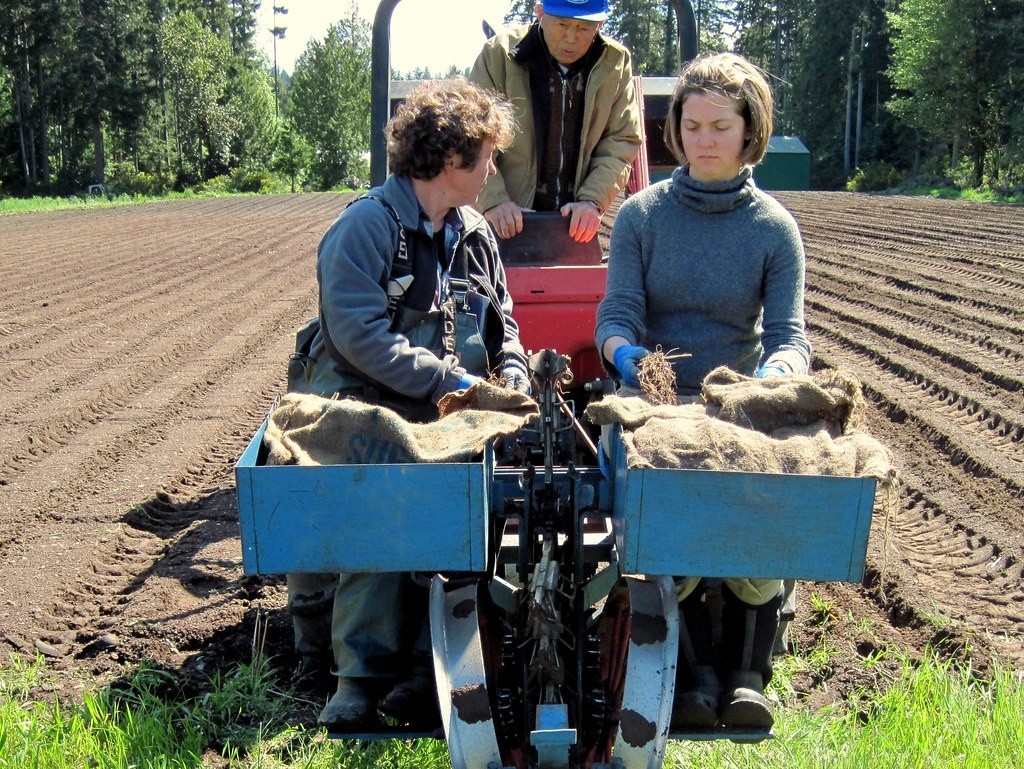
[669,577,720,733]
[715,581,785,743]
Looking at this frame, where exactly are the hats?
[540,0,609,22]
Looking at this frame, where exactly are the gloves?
[756,364,786,379]
[454,373,479,390]
[613,345,653,391]
[500,366,532,396]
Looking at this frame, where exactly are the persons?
[595,53,811,744]
[290,82,532,728]
[468,0,642,243]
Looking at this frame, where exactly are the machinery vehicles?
[234,0,880,769]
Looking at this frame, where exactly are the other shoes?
[378,669,443,732]
[316,676,378,733]
